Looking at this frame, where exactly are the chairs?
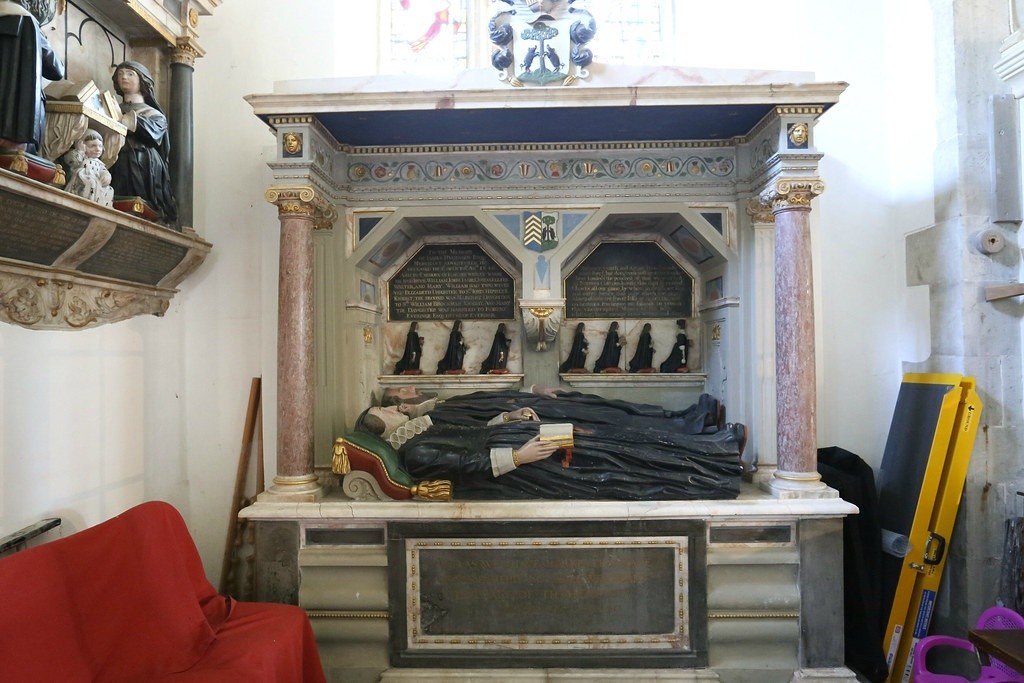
[913,608,1024,683]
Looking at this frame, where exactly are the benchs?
[0,499,327,682]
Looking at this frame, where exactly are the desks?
[970,626,1024,675]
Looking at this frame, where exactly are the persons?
[437,320,466,373]
[660,319,689,372]
[394,322,422,374]
[594,321,628,371]
[62,129,114,207]
[350,383,749,500]
[559,323,590,372]
[0,0,65,156]
[112,60,180,231]
[478,321,513,375]
[628,323,655,373]
